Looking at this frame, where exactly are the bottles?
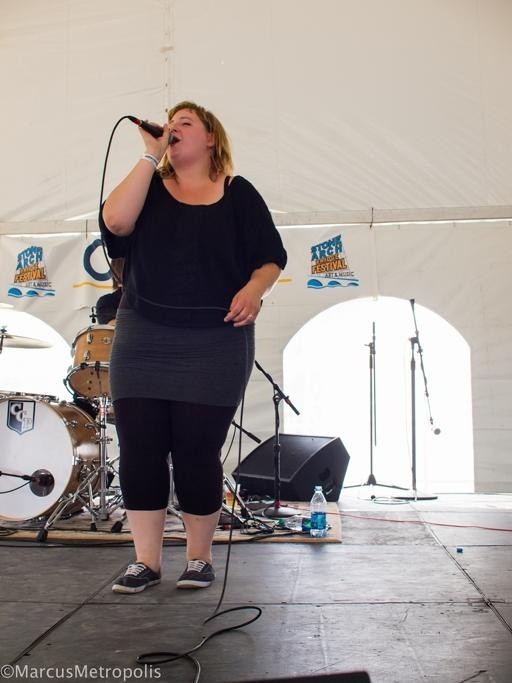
[309,485,329,538]
[277,514,330,534]
[223,482,241,507]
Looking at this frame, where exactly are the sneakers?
[176,558,215,589]
[111,561,161,594]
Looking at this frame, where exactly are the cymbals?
[0,334,48,348]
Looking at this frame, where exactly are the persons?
[73,255,125,491]
[96,100,289,596]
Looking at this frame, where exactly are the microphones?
[22,473,53,487]
[129,115,174,145]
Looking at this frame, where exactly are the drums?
[1,393,182,531]
[66,324,115,398]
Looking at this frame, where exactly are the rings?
[248,314,254,320]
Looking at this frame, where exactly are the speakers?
[231,433,350,502]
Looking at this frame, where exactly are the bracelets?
[140,151,162,171]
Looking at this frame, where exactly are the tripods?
[37,398,246,541]
[343,322,409,490]
